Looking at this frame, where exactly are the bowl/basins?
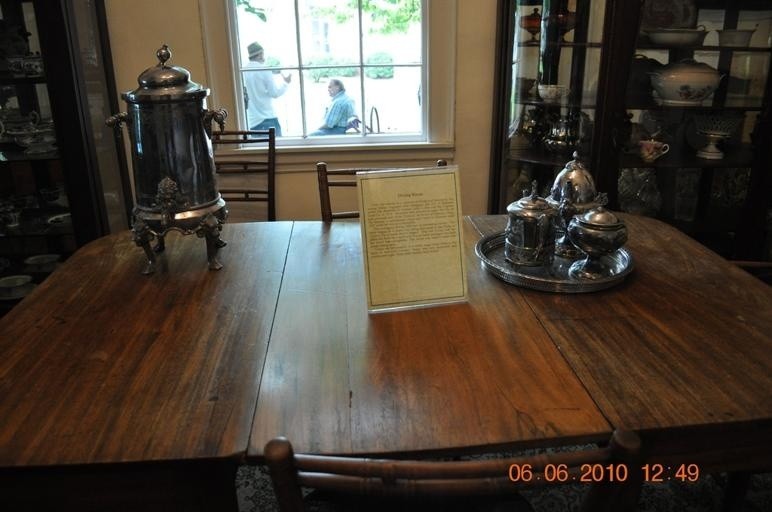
[536,85,565,104]
[716,23,760,49]
[642,26,710,44]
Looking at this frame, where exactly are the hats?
[248,42,264,58]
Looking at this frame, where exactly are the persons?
[242,41,292,138]
[308,80,355,135]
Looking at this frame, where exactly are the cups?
[638,141,670,164]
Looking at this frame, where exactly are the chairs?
[316,160,448,222]
[264,428,643,511]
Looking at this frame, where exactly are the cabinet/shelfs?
[603,0,772,286]
[0,0,102,319]
[487,1,606,214]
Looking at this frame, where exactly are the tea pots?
[504,189,557,267]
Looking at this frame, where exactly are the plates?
[47,212,72,228]
